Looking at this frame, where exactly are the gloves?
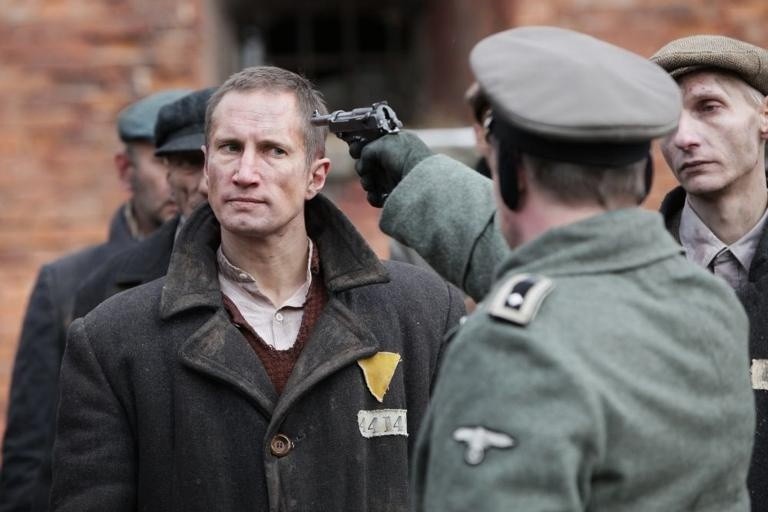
[349,131,435,208]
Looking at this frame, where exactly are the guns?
[308,98,404,201]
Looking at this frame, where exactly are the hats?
[116,88,194,147]
[463,26,683,167]
[647,34,768,97]
[155,88,220,157]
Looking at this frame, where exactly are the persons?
[35,88,219,510]
[49,66,471,510]
[644,28,767,509]
[347,23,756,511]
[384,73,510,321]
[1,88,196,510]
